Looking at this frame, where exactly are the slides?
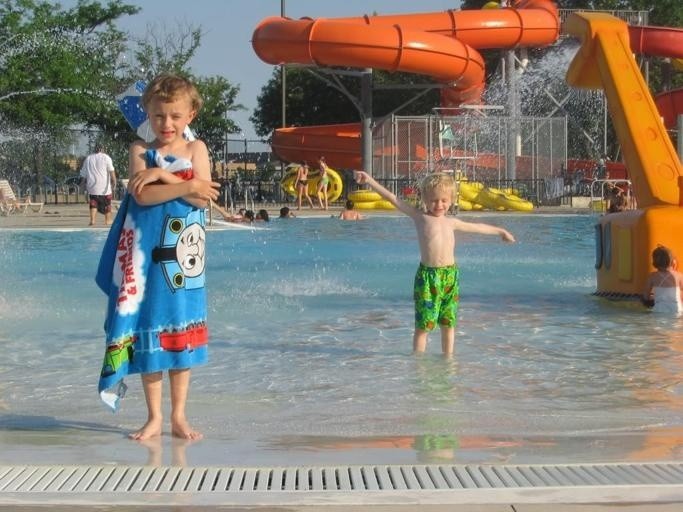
[253,0,630,181]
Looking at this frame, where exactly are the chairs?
[0,179,45,215]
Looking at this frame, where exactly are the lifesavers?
[376,200,396,208]
[455,177,534,211]
[348,190,381,202]
[353,202,378,208]
[283,169,312,198]
[313,168,342,202]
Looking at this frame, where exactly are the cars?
[18,175,86,194]
[252,190,274,202]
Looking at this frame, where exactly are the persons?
[278,207,294,218]
[77,142,118,227]
[243,209,254,224]
[350,167,517,354]
[254,209,269,222]
[600,182,631,215]
[293,159,314,210]
[90,69,221,442]
[336,199,363,221]
[641,245,682,316]
[315,154,330,210]
